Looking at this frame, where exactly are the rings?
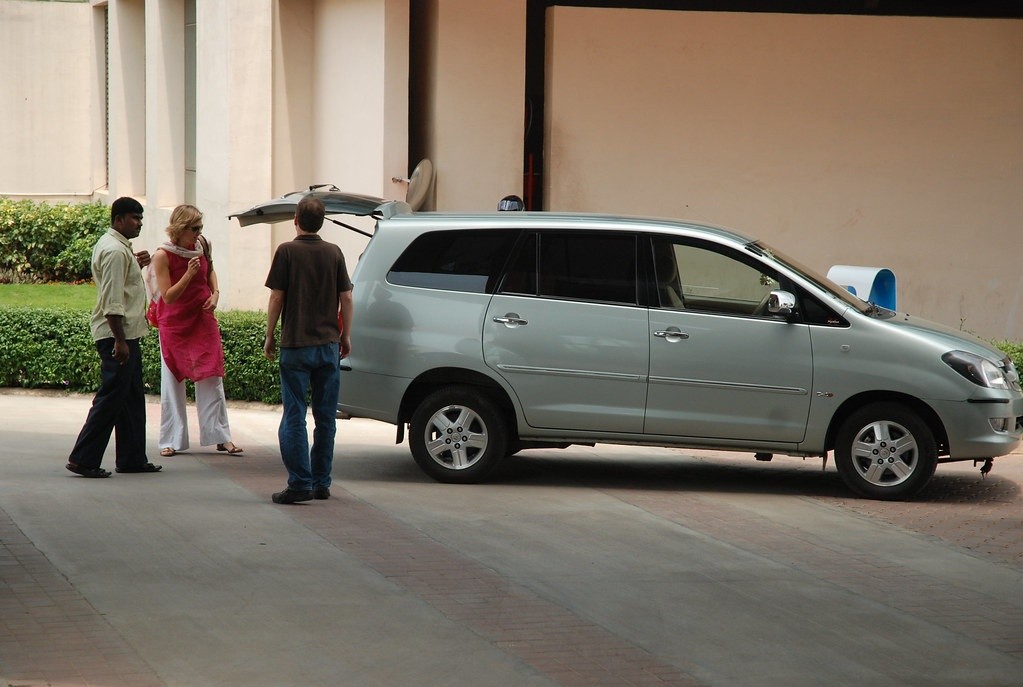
[193,265,198,268]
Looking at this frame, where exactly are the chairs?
[656,255,686,310]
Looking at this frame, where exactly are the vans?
[229,182,1022,503]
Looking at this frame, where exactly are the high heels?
[160,446,175,456]
[216,441,244,453]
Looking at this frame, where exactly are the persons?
[264,194,353,503]
[66,197,243,478]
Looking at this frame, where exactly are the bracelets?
[178,281,186,292]
[216,290,220,293]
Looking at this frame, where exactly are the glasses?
[187,224,203,232]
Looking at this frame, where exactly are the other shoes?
[271,486,312,504]
[313,486,330,500]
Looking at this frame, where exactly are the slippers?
[115,463,162,473]
[66,463,112,478]
